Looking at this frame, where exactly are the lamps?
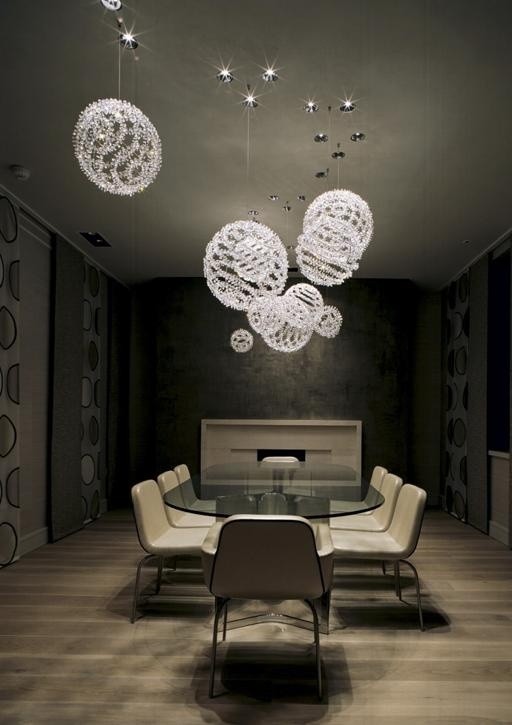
[70,14,161,196]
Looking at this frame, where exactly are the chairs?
[261,455,298,464]
[157,470,213,571]
[174,464,216,513]
[330,472,403,575]
[199,514,334,697]
[129,478,203,622]
[332,483,428,633]
[331,465,388,517]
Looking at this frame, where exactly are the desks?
[164,461,384,519]
[200,418,362,486]
